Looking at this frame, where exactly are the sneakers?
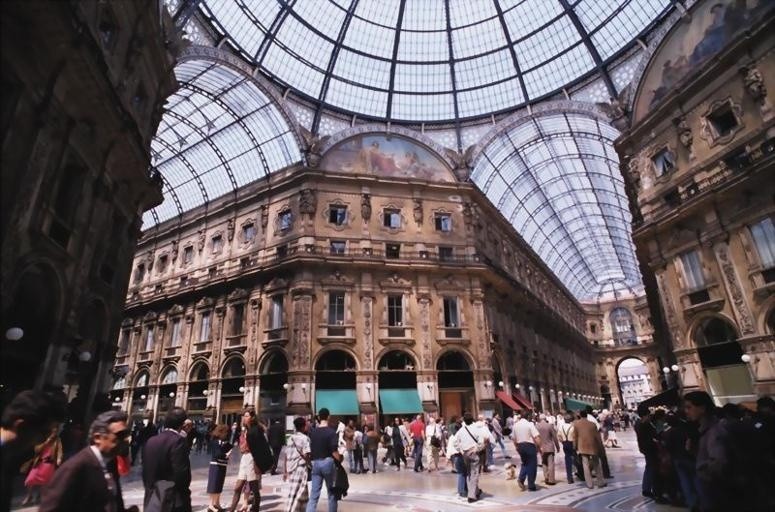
[567,480,574,484]
[642,490,688,508]
[528,486,536,491]
[382,458,443,472]
[575,472,614,489]
[21,495,40,507]
[348,465,378,474]
[468,489,482,503]
[545,478,556,485]
[517,478,526,490]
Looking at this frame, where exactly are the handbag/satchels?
[306,460,314,481]
[431,436,441,448]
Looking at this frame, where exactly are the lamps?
[672,365,686,373]
[495,379,605,401]
[1,325,24,345]
[114,382,291,402]
[740,353,761,365]
[62,351,91,365]
[662,366,671,374]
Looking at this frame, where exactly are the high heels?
[207,502,259,512]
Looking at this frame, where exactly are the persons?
[405,152,434,178]
[360,139,398,176]
[649,0,753,104]
[739,64,770,119]
